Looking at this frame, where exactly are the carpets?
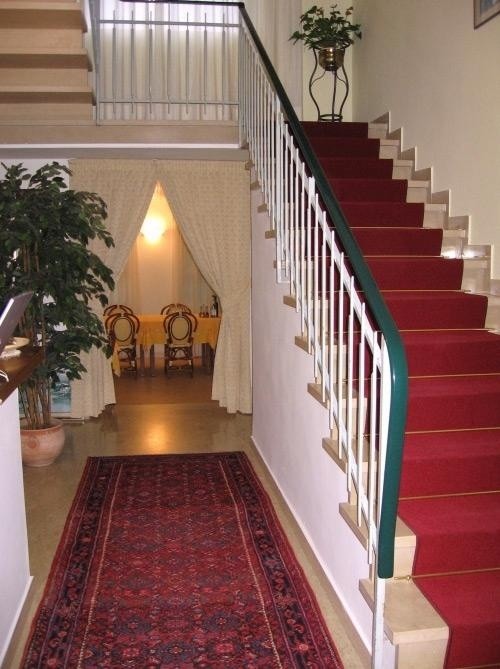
[18,452,351,668]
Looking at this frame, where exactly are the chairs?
[103,304,198,378]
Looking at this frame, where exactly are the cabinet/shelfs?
[309,39,350,123]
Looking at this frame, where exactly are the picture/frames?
[473,0,500,29]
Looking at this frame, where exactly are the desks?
[101,313,221,377]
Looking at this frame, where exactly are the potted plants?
[0,159,116,469]
[288,4,363,70]
